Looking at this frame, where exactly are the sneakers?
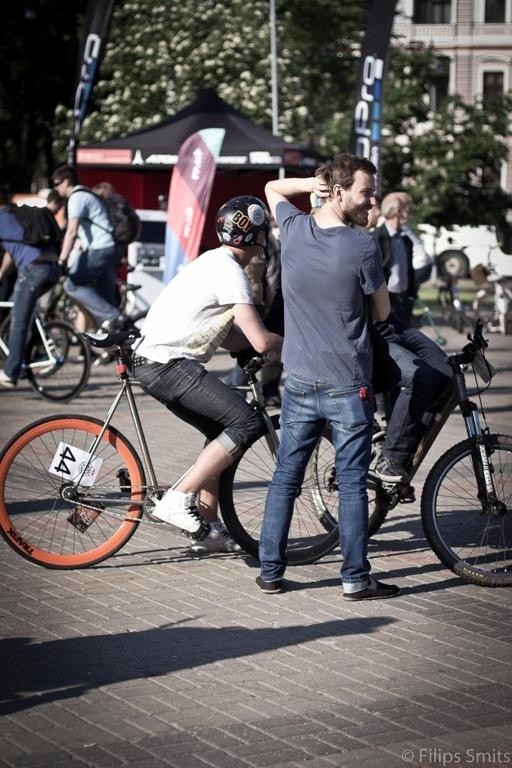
[151,489,211,536]
[373,454,407,483]
[0,369,17,390]
[341,577,400,602]
[189,519,248,553]
[89,307,132,355]
[255,575,285,594]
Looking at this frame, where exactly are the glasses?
[51,177,67,187]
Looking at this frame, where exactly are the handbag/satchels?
[385,236,408,292]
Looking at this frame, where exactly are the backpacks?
[0,203,62,248]
[66,188,142,246]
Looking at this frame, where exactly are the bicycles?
[0,325,342,593]
[300,314,512,587]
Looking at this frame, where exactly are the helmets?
[214,195,270,247]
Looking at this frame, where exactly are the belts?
[131,357,156,368]
